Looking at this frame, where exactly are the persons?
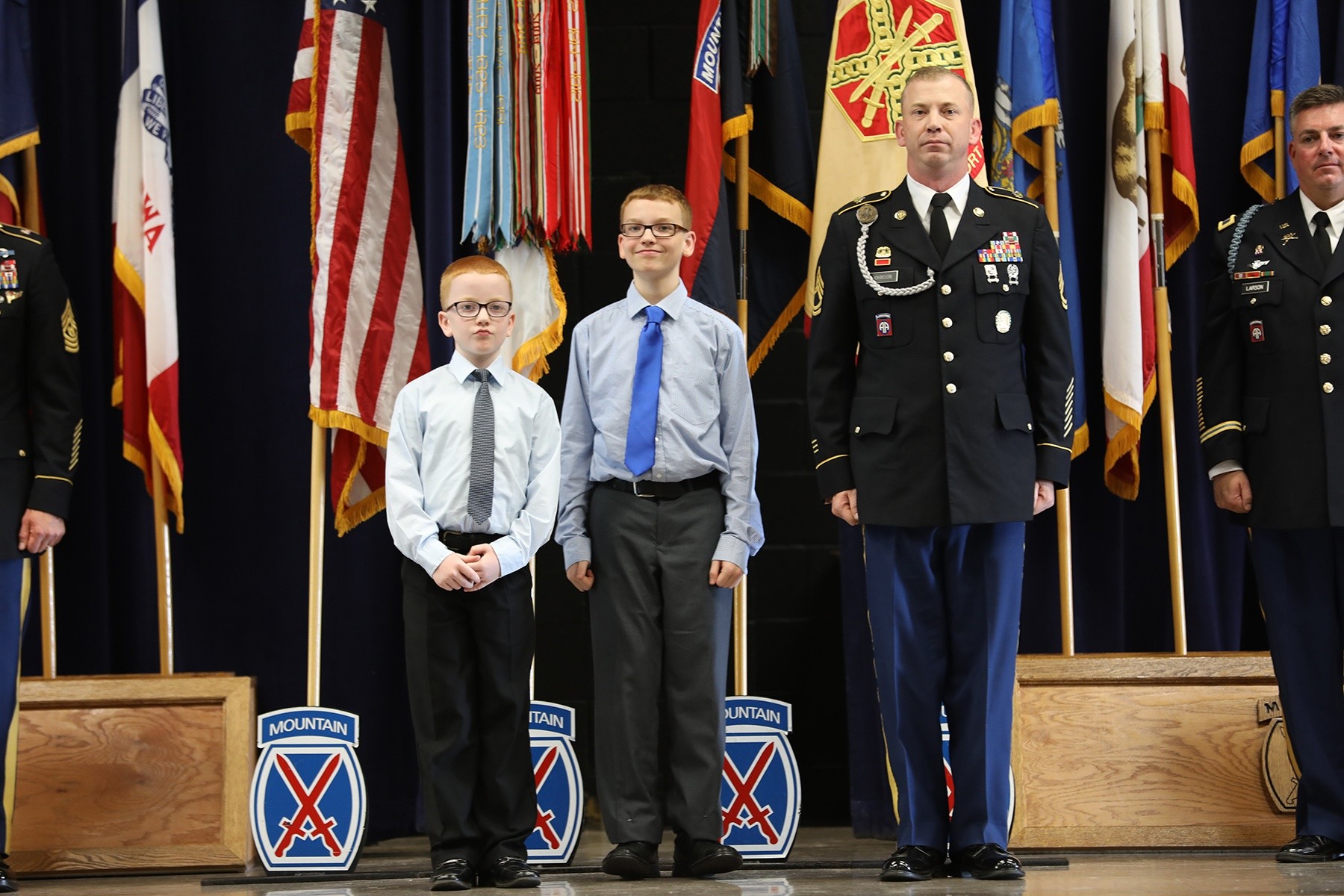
[556,182,762,878]
[100,0,193,536]
[0,220,78,885]
[808,66,1077,879]
[1195,85,1344,862]
[382,253,563,888]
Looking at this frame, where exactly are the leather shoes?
[674,839,743,879]
[1276,834,1344,862]
[879,845,945,879]
[0,866,19,892]
[601,842,661,879]
[429,858,473,890]
[948,839,1025,879]
[473,855,541,888]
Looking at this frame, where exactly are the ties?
[1310,212,1333,280]
[929,193,952,260]
[468,368,495,526]
[624,306,666,476]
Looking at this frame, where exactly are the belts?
[596,470,722,496]
[437,530,509,551]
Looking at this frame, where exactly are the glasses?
[444,301,513,318]
[620,223,689,238]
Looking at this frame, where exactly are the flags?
[494,243,568,386]
[286,0,438,538]
[677,2,754,329]
[1236,0,1325,204]
[1097,0,1202,502]
[806,0,988,331]
[0,0,44,228]
[990,0,1090,462]
